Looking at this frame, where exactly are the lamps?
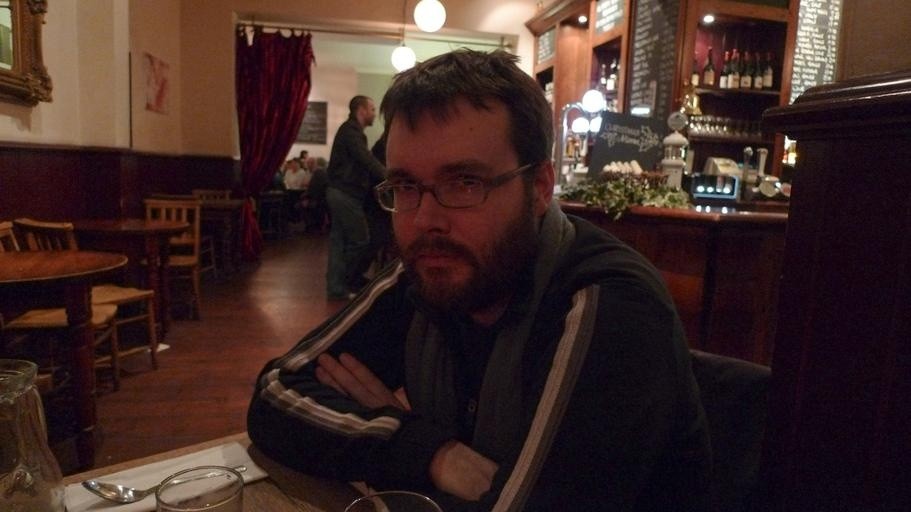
[412,0,446,32]
[390,0,414,72]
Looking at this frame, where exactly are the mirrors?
[0,0,54,104]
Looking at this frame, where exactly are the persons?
[268,149,332,241]
[246,47,714,512]
[324,96,388,300]
[351,134,387,294]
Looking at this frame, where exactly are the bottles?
[702,46,715,88]
[718,48,778,89]
[596,57,620,90]
[690,50,700,88]
[564,127,596,159]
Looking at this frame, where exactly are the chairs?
[685,345,775,510]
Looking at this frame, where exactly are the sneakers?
[328,290,356,302]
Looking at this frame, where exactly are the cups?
[153,466,246,512]
[341,490,440,512]
[686,114,773,142]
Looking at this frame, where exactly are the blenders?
[660,130,688,193]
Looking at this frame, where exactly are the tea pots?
[0,356,65,511]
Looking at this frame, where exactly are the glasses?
[371,162,534,213]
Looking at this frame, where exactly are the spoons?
[82,466,248,508]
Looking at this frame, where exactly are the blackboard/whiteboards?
[627,0,680,121]
[587,111,668,181]
[787,0,844,106]
[593,1,627,41]
[534,23,558,64]
